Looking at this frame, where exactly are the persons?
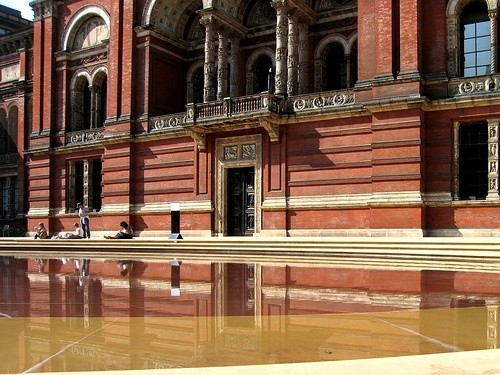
[77,259,91,291]
[34,222,47,239]
[76,203,91,238]
[61,258,80,275]
[103,220,134,240]
[117,260,134,276]
[59,222,83,239]
[35,258,47,273]
